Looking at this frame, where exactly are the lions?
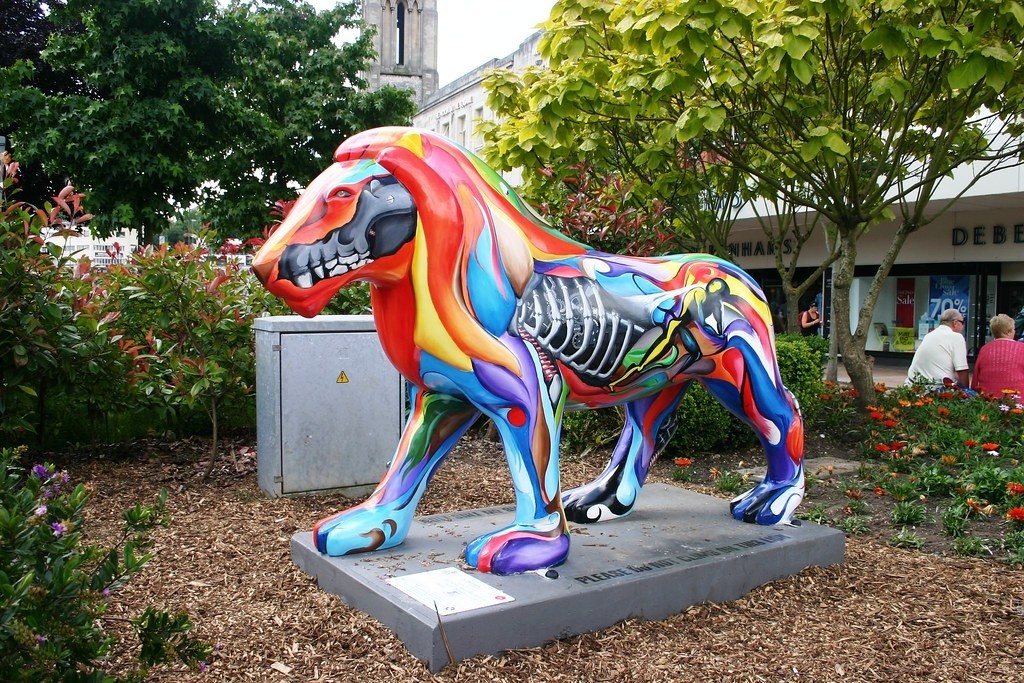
[252,131,802,579]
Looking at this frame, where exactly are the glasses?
[953,319,964,325]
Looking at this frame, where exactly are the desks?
[888,326,914,351]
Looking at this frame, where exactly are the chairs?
[873,320,907,351]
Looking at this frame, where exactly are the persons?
[972,314,1024,406]
[801,303,822,338]
[905,308,970,394]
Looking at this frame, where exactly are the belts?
[908,378,944,385]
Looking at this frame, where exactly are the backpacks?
[798,311,819,326]
